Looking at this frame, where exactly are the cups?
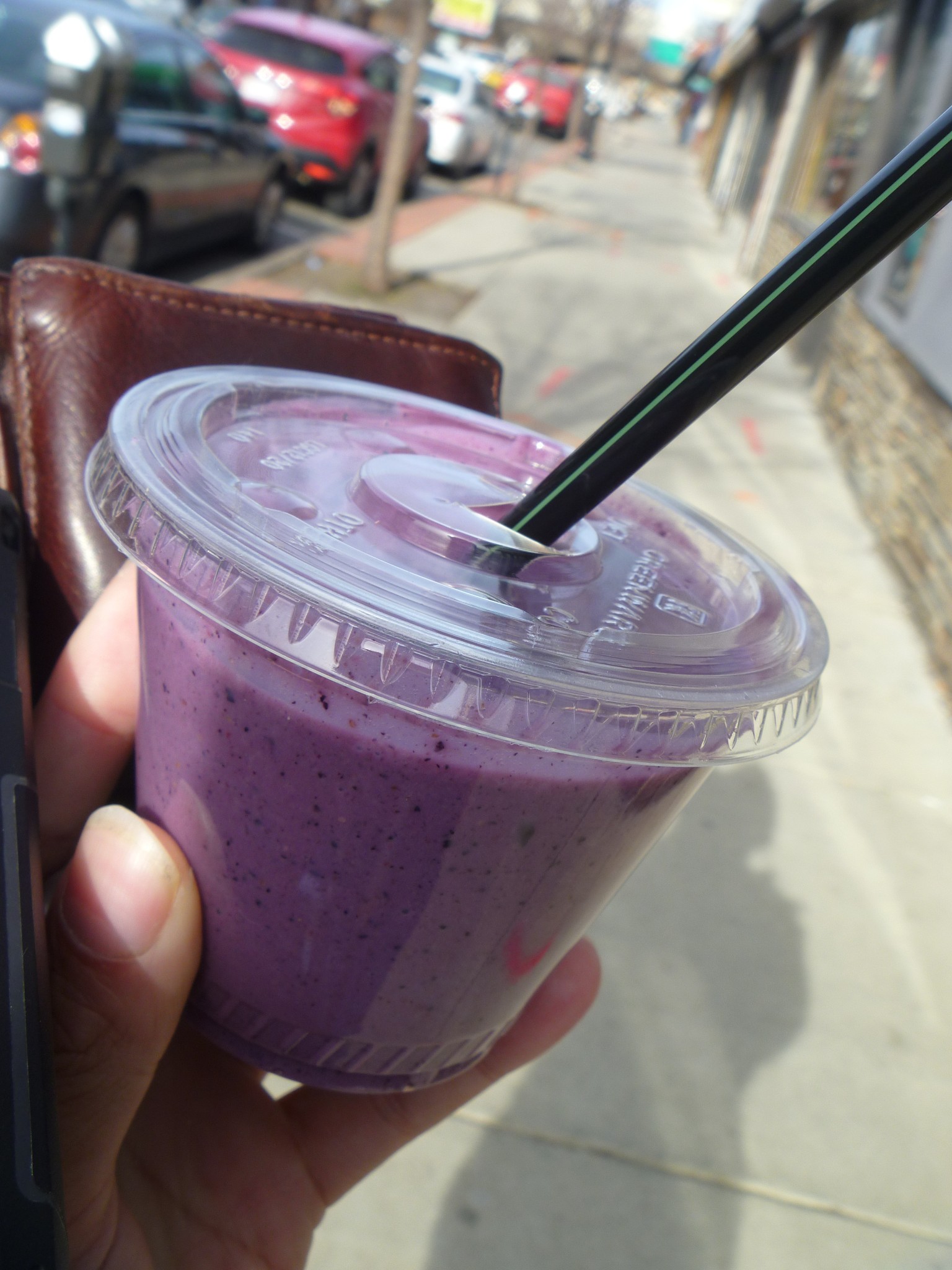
[85,357,828,1091]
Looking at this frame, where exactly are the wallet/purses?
[11,259,501,818]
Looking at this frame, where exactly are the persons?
[0,554,600,1270]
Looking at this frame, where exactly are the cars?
[397,36,685,177]
[0,0,290,278]
[192,6,430,216]
